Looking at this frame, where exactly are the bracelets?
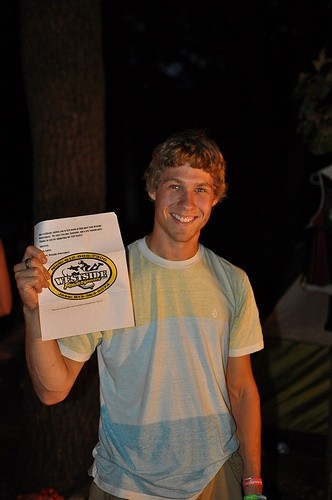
[243,495,267,499]
[241,476,263,488]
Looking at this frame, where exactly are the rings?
[24,258,32,270]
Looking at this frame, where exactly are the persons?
[11,130,269,500]
